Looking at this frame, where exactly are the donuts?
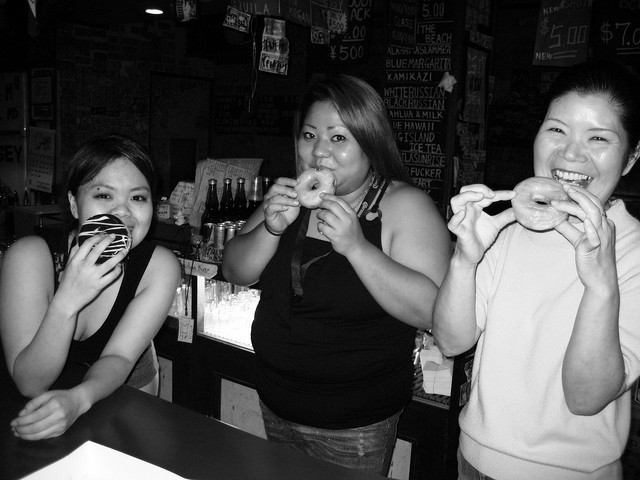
[511,176,569,231]
[79,214,131,262]
[294,168,335,209]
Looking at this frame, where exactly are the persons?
[434,59,640,476]
[0,132,185,444]
[221,71,450,471]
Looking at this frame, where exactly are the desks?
[0,385,377,476]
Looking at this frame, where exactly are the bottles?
[263,178,272,194]
[246,177,263,219]
[200,178,221,234]
[232,178,246,219]
[218,177,233,222]
